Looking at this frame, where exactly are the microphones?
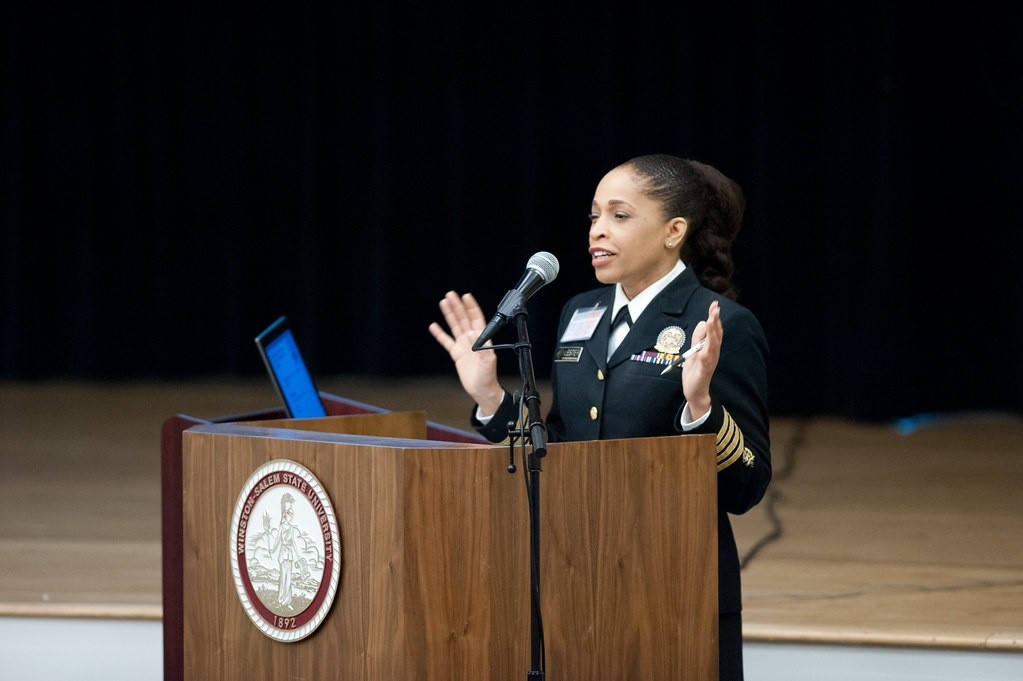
[471,251,560,351]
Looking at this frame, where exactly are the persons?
[429,154,772,680]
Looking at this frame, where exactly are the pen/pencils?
[660,337,706,376]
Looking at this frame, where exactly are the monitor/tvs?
[254,316,329,418]
